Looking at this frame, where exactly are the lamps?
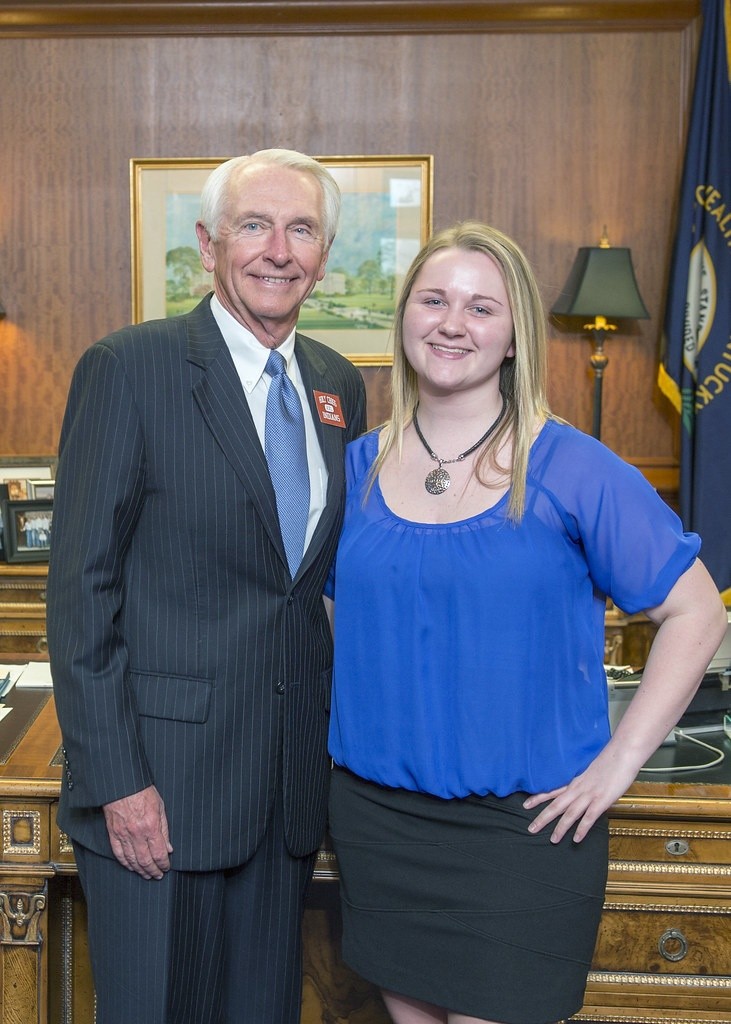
[549,225,651,440]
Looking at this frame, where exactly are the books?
[15,661,53,687]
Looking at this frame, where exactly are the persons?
[46,148,368,1024]
[20,514,49,547]
[328,222,728,1023]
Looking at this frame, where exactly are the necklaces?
[412,390,507,495]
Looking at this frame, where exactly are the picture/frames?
[0,484,10,562]
[2,499,53,563]
[0,455,59,500]
[129,154,433,367]
[26,478,55,499]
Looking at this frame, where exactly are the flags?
[654,0,731,610]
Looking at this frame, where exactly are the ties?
[263,349,311,581]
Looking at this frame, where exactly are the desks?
[0,661,731,1024]
